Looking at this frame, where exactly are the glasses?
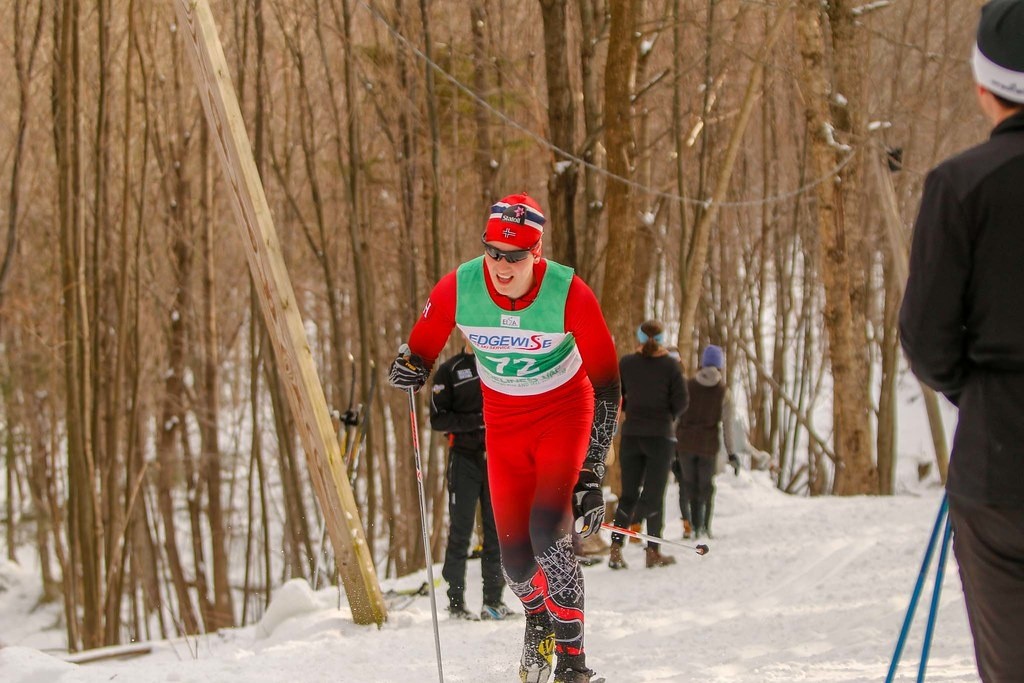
[481,231,542,263]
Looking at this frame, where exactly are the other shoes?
[448,605,479,622]
[702,526,713,539]
[694,526,700,539]
[481,602,523,622]
[684,520,691,533]
[571,535,612,557]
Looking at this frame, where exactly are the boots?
[644,548,676,568]
[608,541,626,569]
[629,524,642,543]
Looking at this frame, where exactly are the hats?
[484,191,546,259]
[701,345,722,369]
[971,0,1024,104]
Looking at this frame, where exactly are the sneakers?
[519,610,555,683]
[554,668,596,683]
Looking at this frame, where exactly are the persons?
[429,330,523,621]
[388,191,622,683]
[899,0,1024,683]
[572,320,740,570]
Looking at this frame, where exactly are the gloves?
[388,352,432,395]
[729,454,740,476]
[571,470,606,538]
